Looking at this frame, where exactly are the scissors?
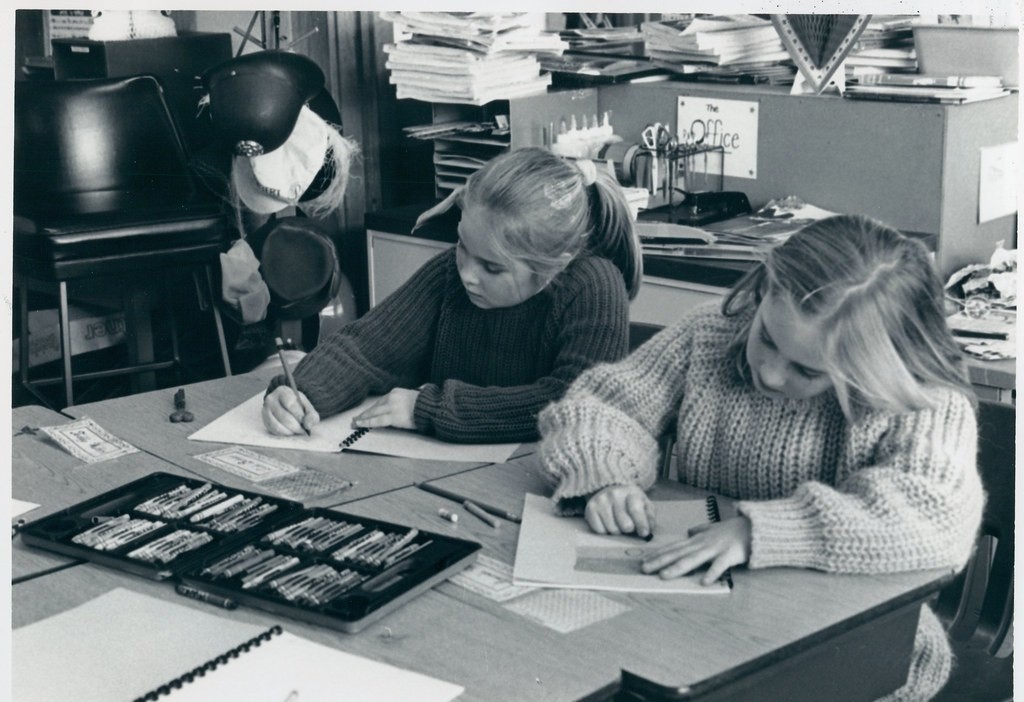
[638,120,673,200]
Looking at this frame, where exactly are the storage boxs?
[51,31,234,83]
[911,26,1021,80]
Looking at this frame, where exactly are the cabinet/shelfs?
[367,80,1017,581]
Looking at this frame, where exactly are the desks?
[11,363,952,702]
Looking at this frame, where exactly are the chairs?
[16,75,241,411]
[936,399,1013,702]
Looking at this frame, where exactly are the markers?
[13,519,28,537]
[71,479,438,609]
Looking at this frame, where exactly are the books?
[13,586,464,702]
[189,388,520,464]
[510,492,735,593]
[381,10,1011,261]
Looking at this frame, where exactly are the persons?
[538,215,984,702]
[262,148,643,445]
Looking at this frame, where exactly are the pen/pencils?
[276,337,313,436]
[413,480,521,524]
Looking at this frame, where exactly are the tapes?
[621,143,638,182]
